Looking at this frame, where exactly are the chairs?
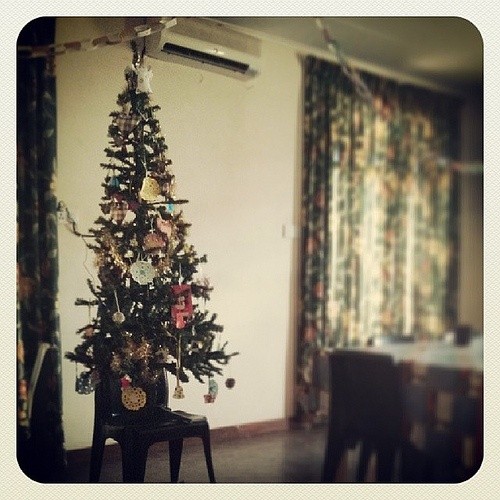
[396,360,484,484]
[90,338,215,483]
[323,350,395,483]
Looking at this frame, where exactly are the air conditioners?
[144,17,264,83]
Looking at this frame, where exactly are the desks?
[367,334,484,372]
